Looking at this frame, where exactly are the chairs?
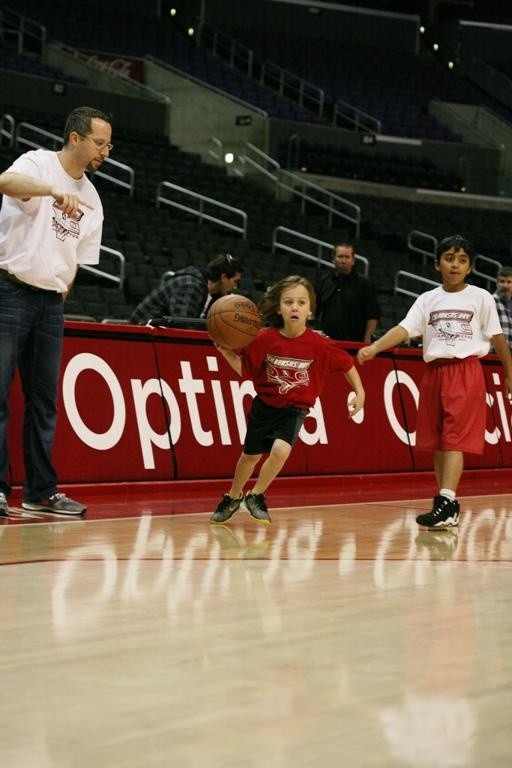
[0,0,512,352]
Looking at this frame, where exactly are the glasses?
[75,130,112,151]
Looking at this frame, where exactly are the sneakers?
[1,491,10,514]
[240,489,272,526]
[210,490,242,524]
[416,495,459,528]
[22,493,86,514]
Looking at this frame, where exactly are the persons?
[490,266,512,354]
[208,274,366,525]
[356,232,512,530]
[211,523,282,637]
[379,526,475,768]
[315,240,381,355]
[0,105,113,518]
[127,251,245,325]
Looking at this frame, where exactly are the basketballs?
[206,294,261,348]
